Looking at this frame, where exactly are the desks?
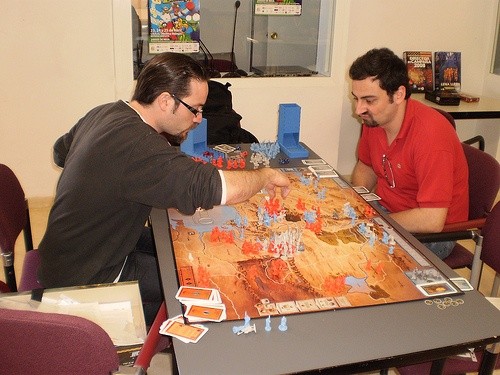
[149,142,500,375]
[408,93,500,118]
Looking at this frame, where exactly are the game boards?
[165,166,466,326]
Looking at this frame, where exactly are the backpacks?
[201,80,243,145]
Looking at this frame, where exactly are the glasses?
[382,155,395,189]
[169,92,203,117]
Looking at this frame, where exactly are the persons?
[36,49,291,327]
[346,48,470,263]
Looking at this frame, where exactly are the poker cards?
[213,144,236,153]
[352,185,381,202]
[159,286,228,343]
[417,276,474,297]
[301,159,339,178]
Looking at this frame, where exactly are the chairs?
[0,164,168,375]
[386,107,500,375]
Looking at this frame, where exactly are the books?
[434,51,461,94]
[403,51,433,93]
